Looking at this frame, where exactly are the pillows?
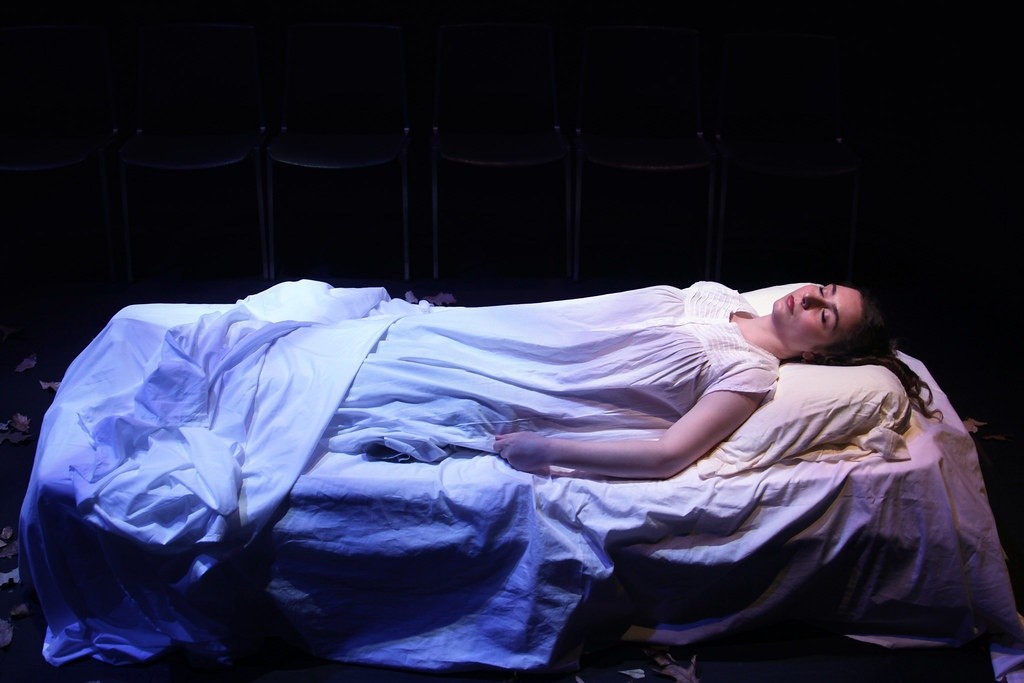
[698,281,912,477]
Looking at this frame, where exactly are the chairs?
[574,23,716,282]
[0,24,126,280]
[714,31,863,286]
[268,22,411,284]
[428,24,572,281]
[116,23,266,282]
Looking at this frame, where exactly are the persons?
[319,278,944,480]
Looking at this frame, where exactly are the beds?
[20,349,1024,683]
